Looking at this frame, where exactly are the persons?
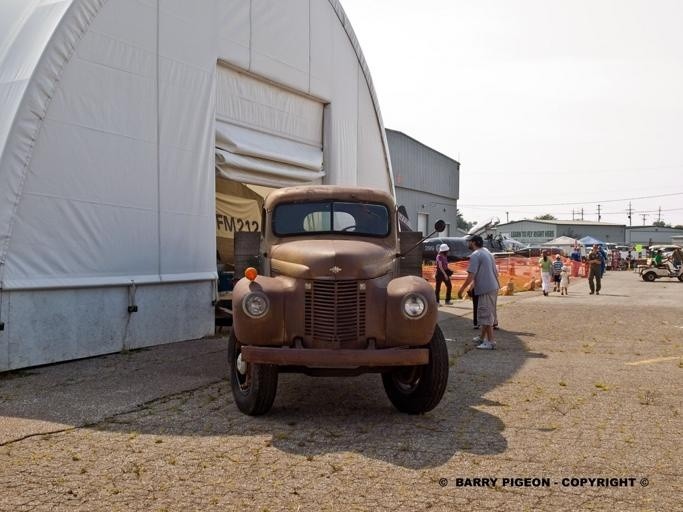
[459,235,501,351]
[434,234,683,329]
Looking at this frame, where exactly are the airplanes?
[398,217,563,264]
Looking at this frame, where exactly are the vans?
[561,244,682,282]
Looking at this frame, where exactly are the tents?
[0,0,402,373]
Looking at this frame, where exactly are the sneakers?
[473,335,484,343]
[476,341,496,350]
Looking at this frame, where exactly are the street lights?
[506,211,509,222]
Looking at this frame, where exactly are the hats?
[440,244,450,251]
[556,254,560,259]
[541,250,549,256]
[466,235,483,247]
[561,266,568,271]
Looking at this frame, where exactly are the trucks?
[227,184,448,417]
